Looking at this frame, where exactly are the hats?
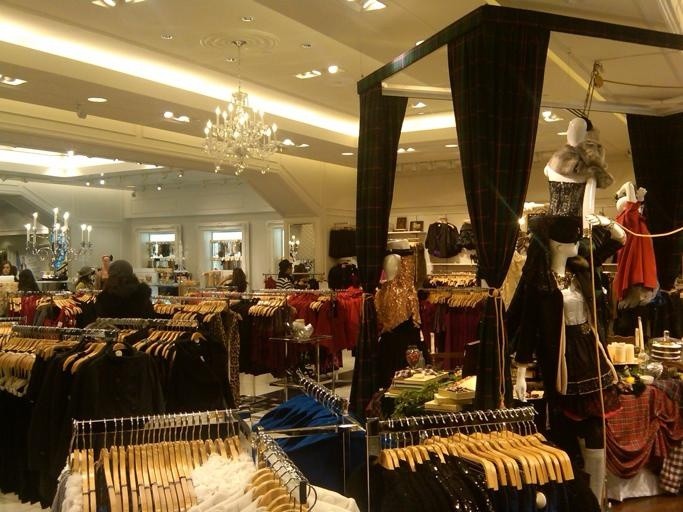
[78,266,95,279]
[279,259,293,267]
[549,217,583,243]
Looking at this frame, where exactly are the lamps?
[22,204,95,272]
[203,43,282,178]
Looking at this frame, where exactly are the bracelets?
[637,201,644,206]
[604,219,614,229]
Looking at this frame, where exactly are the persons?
[276,259,295,289]
[504,213,626,508]
[609,182,664,339]
[9,266,19,282]
[372,238,423,389]
[16,269,40,293]
[74,266,95,292]
[216,267,247,291]
[93,260,153,317]
[541,115,612,221]
[0,260,11,276]
[96,257,110,289]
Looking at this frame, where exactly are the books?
[384,366,474,412]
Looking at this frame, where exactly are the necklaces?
[551,269,571,291]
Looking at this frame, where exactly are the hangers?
[374,406,576,490]
[57,405,319,511]
[426,284,489,310]
